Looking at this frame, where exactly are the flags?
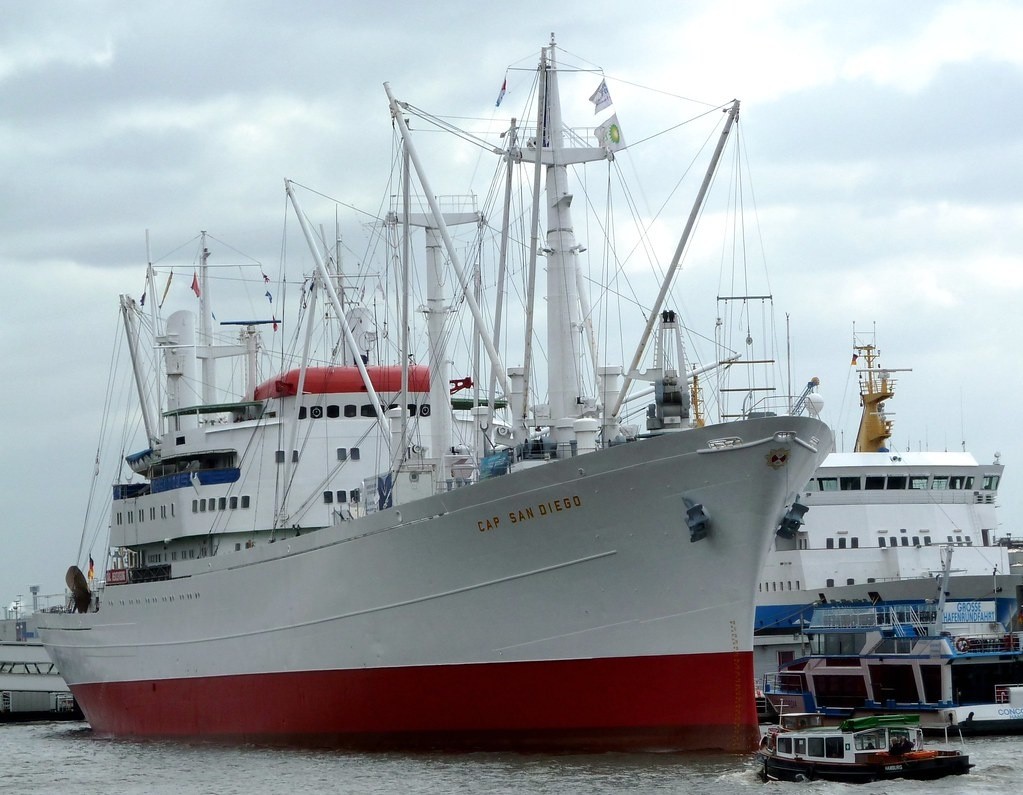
[589,78,612,115]
[273,317,278,332]
[88,557,95,579]
[265,291,272,303]
[191,273,199,297]
[262,272,270,283]
[495,79,506,107]
[594,113,626,152]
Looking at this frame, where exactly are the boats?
[748,319,1023,724]
[754,538,1023,739]
[0,585,86,725]
[754,709,976,784]
[30,32,839,759]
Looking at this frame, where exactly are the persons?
[865,743,874,749]
[900,736,914,753]
[233,415,245,424]
[890,737,904,754]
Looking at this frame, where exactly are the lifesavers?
[956,637,970,652]
[771,733,777,750]
